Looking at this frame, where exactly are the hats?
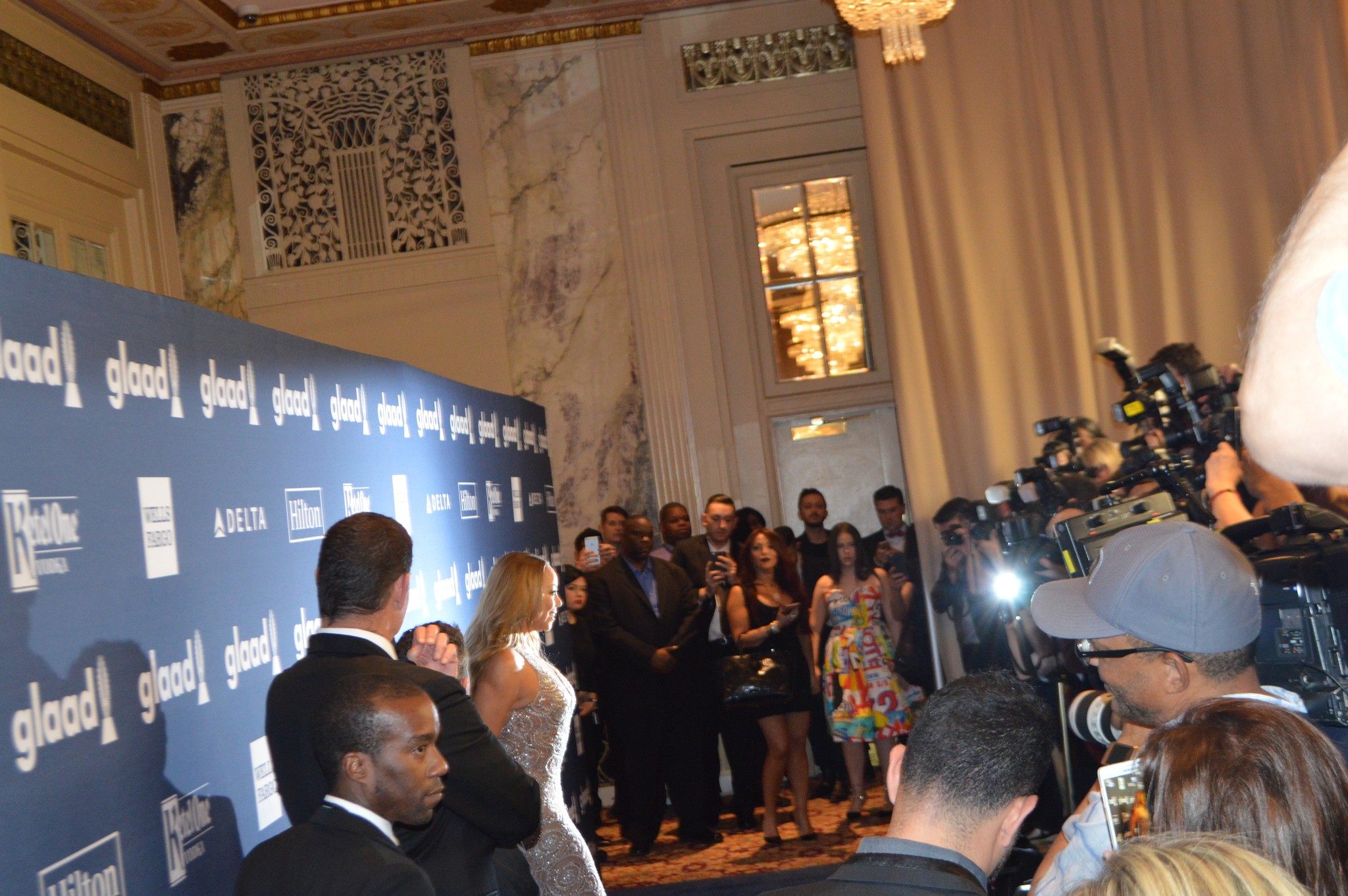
[1029,522,1261,653]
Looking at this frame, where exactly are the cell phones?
[584,536,600,565]
[1096,757,1150,847]
[781,602,800,615]
[877,540,889,551]
[708,551,727,579]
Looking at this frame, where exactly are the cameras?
[941,336,1241,548]
[1065,687,1125,746]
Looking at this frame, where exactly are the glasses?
[1073,639,1195,670]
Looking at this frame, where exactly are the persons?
[231,673,452,896]
[540,342,1348,896]
[393,620,540,896]
[264,512,541,896]
[463,552,607,896]
